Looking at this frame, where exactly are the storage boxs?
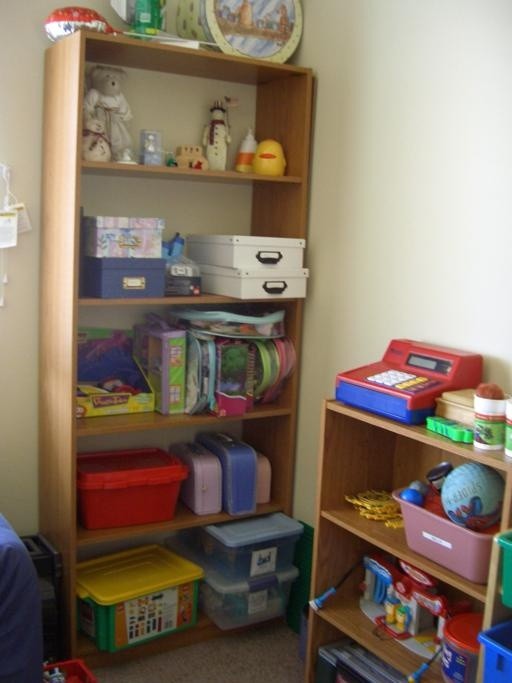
[74,442,193,533]
[40,657,100,682]
[68,541,205,651]
[160,533,301,638]
[193,263,310,301]
[492,531,511,611]
[187,231,308,271]
[79,207,167,261]
[475,617,512,683]
[81,255,171,302]
[177,512,304,581]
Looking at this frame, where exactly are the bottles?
[237,129,258,172]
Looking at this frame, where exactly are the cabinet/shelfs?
[34,25,320,663]
[468,530,511,683]
[300,391,512,683]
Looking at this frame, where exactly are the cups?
[472,392,511,457]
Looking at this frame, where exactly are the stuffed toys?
[201,98,232,171]
[81,62,136,163]
[80,117,113,163]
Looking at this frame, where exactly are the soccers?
[442,462,505,530]
